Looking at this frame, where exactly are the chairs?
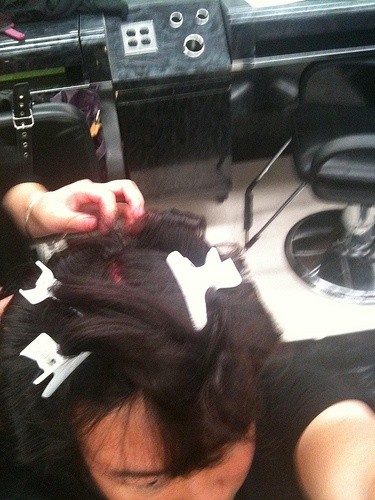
[0,98,125,192]
[242,56,375,307]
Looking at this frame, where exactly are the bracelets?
[25,191,68,246]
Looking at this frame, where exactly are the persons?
[0,209,374,500]
[0,145,145,319]
